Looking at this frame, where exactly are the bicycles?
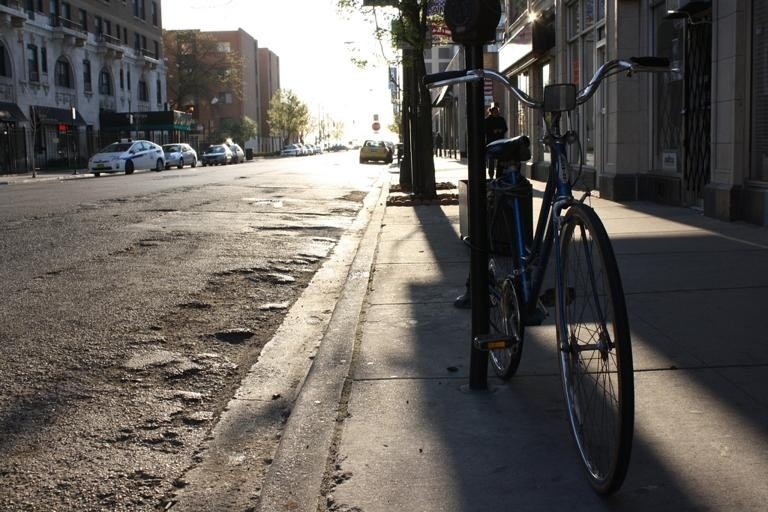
[422,57,681,497]
[281,143,321,156]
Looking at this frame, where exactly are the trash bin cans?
[246,149,252,160]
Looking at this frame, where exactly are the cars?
[202,144,244,167]
[360,140,394,163]
[88,138,166,177]
[162,143,197,170]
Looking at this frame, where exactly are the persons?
[487,106,508,179]
[435,132,443,158]
